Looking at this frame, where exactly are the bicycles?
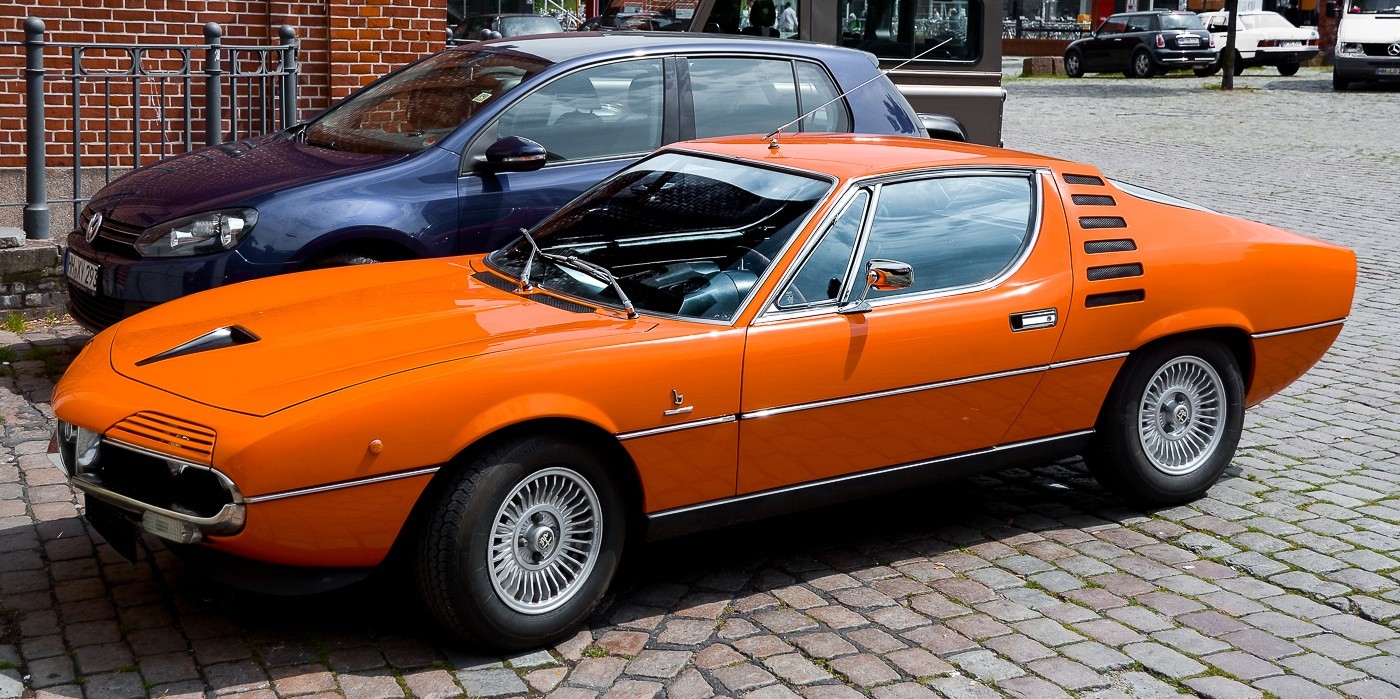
[920,16,1084,39]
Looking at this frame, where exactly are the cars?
[62,30,933,336]
[1155,11,1320,77]
[452,13,565,46]
[1063,11,1216,78]
[576,14,678,32]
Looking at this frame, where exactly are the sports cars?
[43,38,1351,645]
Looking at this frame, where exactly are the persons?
[858,3,869,35]
[948,8,960,38]
[957,8,967,38]
[740,5,750,29]
[748,0,755,17]
[848,12,857,30]
[778,1,798,33]
[1282,0,1301,28]
[769,5,778,30]
[932,7,941,36]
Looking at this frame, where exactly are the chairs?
[605,72,662,156]
[545,74,606,154]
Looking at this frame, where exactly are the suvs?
[499,0,1008,152]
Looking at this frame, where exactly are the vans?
[1333,0,1400,88]
